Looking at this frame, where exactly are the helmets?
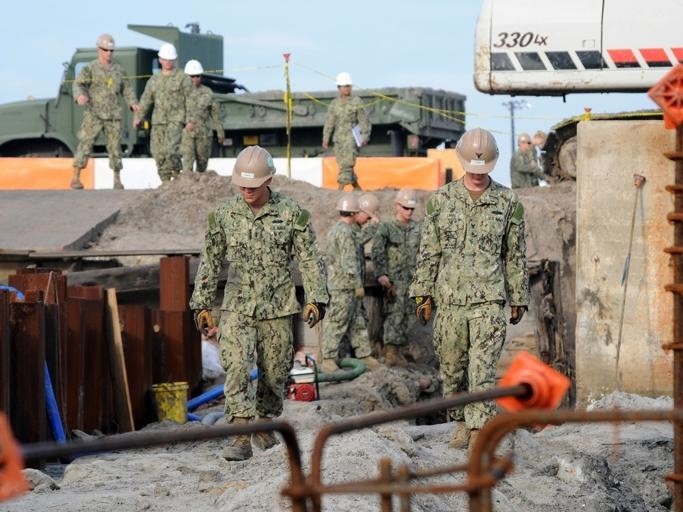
[231,145,276,189]
[454,128,500,174]
[97,34,114,51]
[184,60,204,76]
[158,43,178,60]
[336,193,380,218]
[336,72,353,88]
[394,189,417,209]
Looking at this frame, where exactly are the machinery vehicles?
[471,0,682,187]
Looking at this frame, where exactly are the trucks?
[0,21,468,161]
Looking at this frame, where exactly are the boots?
[318,339,398,374]
[449,421,480,459]
[253,416,280,451]
[223,418,253,460]
[113,170,124,189]
[70,166,84,189]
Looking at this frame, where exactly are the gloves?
[194,309,213,335]
[303,304,325,328]
[510,306,525,325]
[416,296,431,325]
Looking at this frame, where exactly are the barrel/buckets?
[152,381,189,424]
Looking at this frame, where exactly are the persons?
[189,145,330,461]
[409,130,532,450]
[70,32,144,190]
[320,71,374,193]
[510,130,547,187]
[322,188,422,374]
[132,42,199,189]
[180,58,226,174]
